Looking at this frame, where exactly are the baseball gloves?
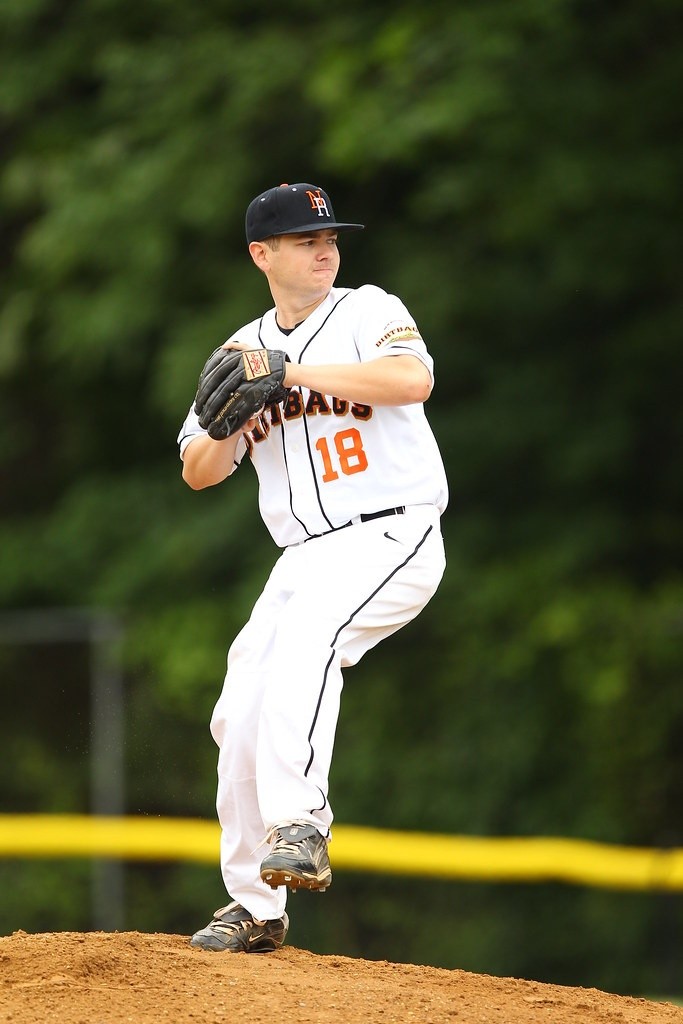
[193,340,294,442]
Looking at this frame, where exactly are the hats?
[245,183,364,245]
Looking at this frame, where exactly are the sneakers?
[190,901,289,953]
[249,821,332,892]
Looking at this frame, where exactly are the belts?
[303,506,405,543]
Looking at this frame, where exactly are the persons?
[177,182,448,954]
